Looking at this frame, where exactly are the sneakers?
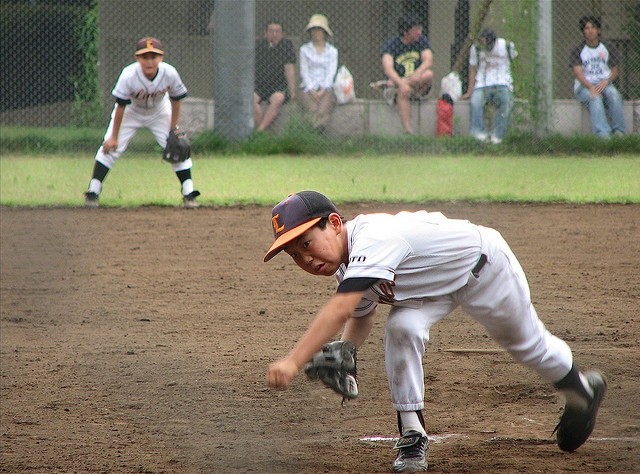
[392,430,430,474]
[550,370,607,451]
[85,191,98,209]
[183,191,201,209]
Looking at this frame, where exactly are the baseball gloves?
[162,128,192,164]
[303,340,359,399]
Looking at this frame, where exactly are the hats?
[303,14,334,37]
[264,191,344,262]
[134,37,164,56]
[476,28,496,50]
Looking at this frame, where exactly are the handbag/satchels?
[436,93,453,139]
[333,65,356,105]
[439,71,463,102]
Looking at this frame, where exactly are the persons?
[263,191,608,473]
[381,12,434,134]
[85,37,202,209]
[297,13,338,133]
[461,28,519,144]
[568,15,627,139]
[252,18,297,130]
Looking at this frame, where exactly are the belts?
[466,254,487,286]
